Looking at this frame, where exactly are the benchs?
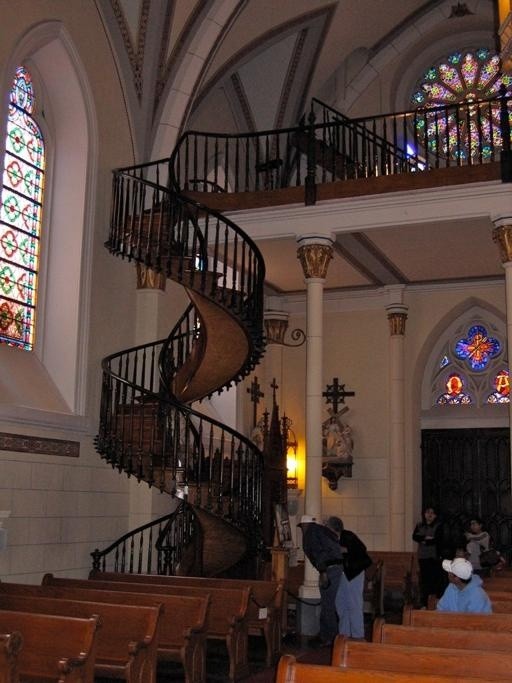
[1,552,419,680]
[275,567,511,683]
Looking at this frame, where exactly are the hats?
[295,515,317,527]
[441,558,473,580]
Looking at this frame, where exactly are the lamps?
[285,430,299,487]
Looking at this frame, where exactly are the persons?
[455,518,491,579]
[435,554,492,612]
[412,503,447,612]
[297,515,344,649]
[324,515,369,643]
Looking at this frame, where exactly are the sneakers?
[415,600,427,609]
[307,638,333,650]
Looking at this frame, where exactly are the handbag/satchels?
[479,549,506,572]
